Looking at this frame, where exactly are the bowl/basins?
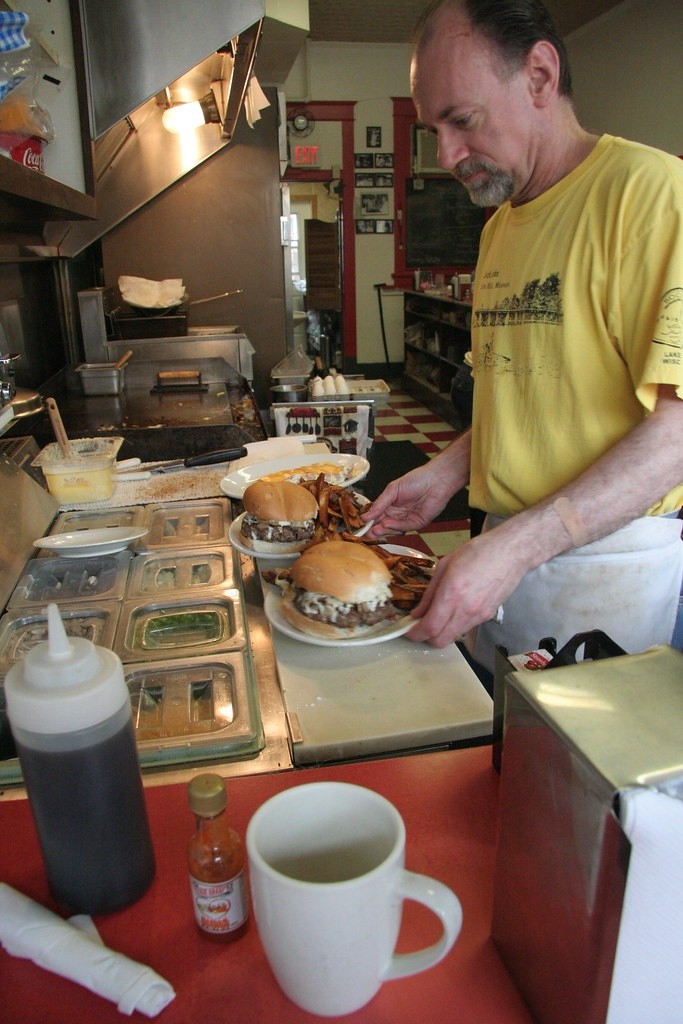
[269,383,308,403]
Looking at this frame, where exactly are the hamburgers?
[275,539,394,640]
[239,481,318,555]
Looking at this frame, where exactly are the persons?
[360,0,683,695]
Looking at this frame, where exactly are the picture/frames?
[353,126,395,234]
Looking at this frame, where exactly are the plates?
[219,453,371,500]
[263,543,438,649]
[228,491,375,560]
[33,525,149,558]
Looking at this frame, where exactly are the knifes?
[116,447,247,475]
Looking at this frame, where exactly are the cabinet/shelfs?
[400,289,476,431]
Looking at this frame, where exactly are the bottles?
[186,773,249,936]
[2,603,156,917]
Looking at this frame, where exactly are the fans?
[286,106,317,138]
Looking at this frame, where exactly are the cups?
[245,779,464,1019]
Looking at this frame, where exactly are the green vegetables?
[145,613,217,643]
